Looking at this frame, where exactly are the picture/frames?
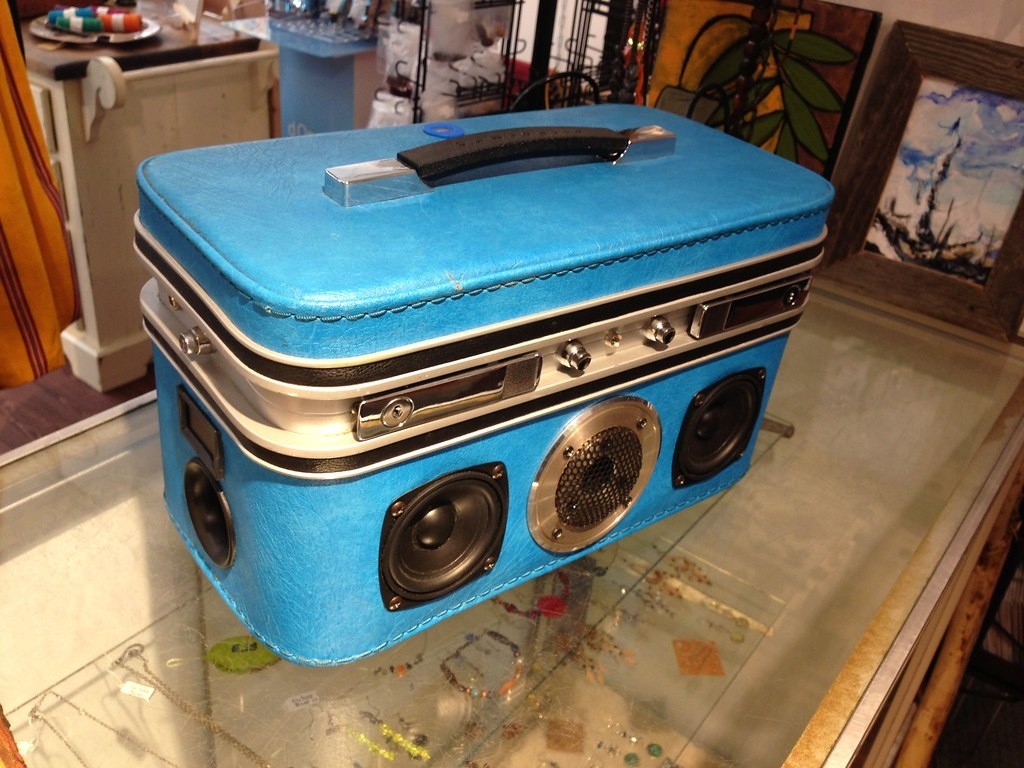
[641,0,885,183]
[814,18,1024,344]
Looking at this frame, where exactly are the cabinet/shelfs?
[20,11,281,394]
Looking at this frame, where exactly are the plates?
[30,12,161,43]
